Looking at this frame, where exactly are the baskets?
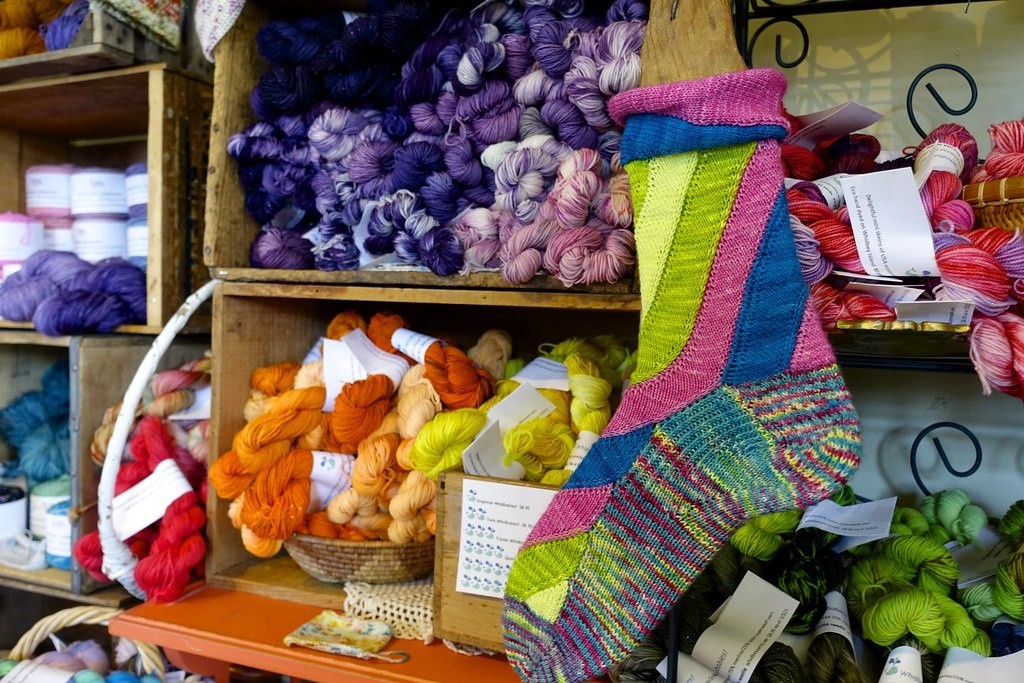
[960,178,1024,235]
[282,533,435,584]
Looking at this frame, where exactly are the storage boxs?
[0,0,640,655]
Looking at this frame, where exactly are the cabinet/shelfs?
[666,0,1024,683]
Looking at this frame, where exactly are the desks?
[108,580,530,683]
